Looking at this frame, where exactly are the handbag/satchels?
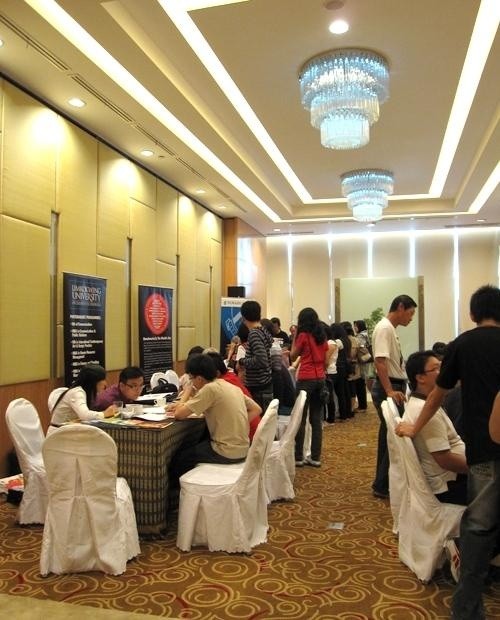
[358,344,373,363]
[319,377,334,405]
[152,378,178,393]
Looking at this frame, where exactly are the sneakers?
[372,481,389,498]
[322,408,366,426]
[443,539,461,583]
[296,458,321,467]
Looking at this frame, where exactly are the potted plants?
[364,307,386,392]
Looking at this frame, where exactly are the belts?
[376,376,409,384]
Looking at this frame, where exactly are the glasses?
[424,367,440,373]
[126,384,145,388]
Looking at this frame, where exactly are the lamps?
[297,47,390,151]
[340,167,394,222]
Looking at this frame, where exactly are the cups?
[113,400,123,417]
[154,397,166,406]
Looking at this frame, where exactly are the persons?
[179,345,219,390]
[290,307,329,467]
[90,366,145,412]
[488,390,500,444]
[205,352,263,447]
[44,364,118,440]
[371,294,418,497]
[321,319,374,429]
[226,300,298,419]
[165,353,251,514]
[401,339,493,506]
[394,284,500,620]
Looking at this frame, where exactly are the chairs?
[177,408,280,554]
[48,387,69,412]
[40,425,141,575]
[381,400,399,534]
[5,398,44,524]
[267,390,308,501]
[396,417,466,580]
[272,406,312,460]
[387,397,400,418]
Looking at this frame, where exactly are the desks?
[79,403,209,537]
